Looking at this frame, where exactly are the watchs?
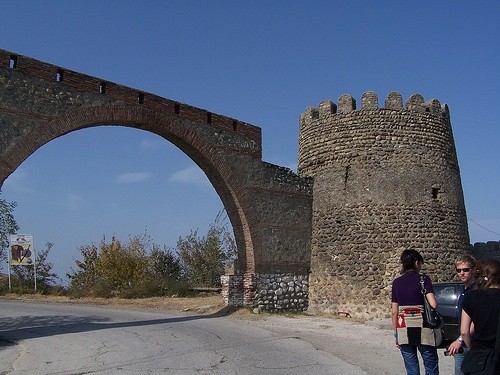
[458,336,464,345]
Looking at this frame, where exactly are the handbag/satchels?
[420,274,445,329]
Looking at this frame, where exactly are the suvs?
[431,282,465,348]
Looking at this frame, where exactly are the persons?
[392,249,439,375]
[448,255,480,375]
[460,260,500,375]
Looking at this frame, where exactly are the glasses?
[455,267,473,272]
